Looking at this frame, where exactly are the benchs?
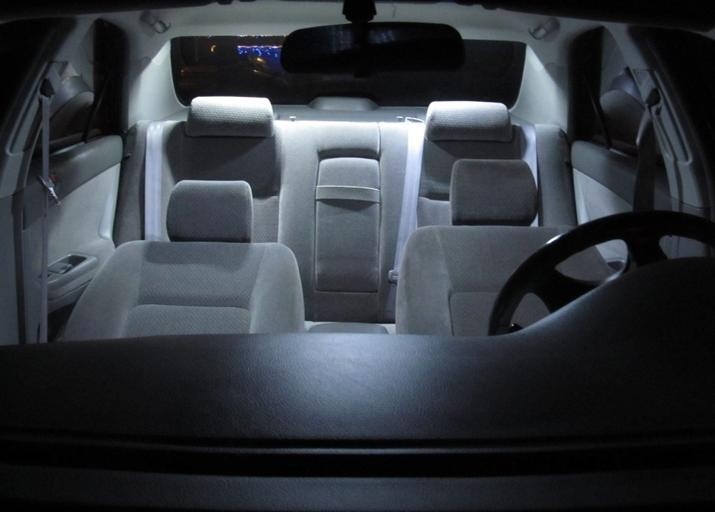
[112,96,579,333]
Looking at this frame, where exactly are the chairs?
[395,158,610,338]
[57,179,304,342]
